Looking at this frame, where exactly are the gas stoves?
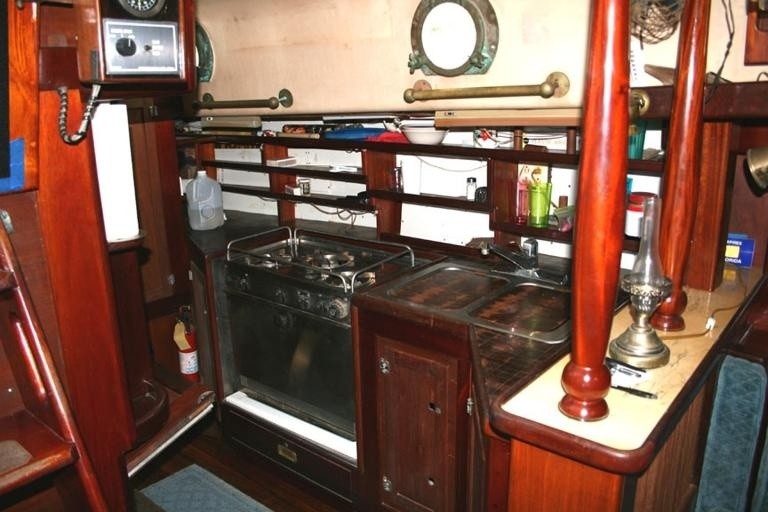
[227,237,418,294]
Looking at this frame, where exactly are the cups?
[505,177,553,230]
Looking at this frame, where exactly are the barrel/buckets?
[186,171,224,232]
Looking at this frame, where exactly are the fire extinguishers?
[176,305,200,380]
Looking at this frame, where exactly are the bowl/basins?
[400,128,449,145]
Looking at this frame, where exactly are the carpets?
[138,462,276,511]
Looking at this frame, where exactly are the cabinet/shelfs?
[350,303,511,511]
[200,132,663,265]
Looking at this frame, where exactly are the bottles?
[465,176,477,202]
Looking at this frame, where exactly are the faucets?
[480,237,539,276]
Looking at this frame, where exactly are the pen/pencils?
[606,357,658,400]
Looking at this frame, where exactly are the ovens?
[227,264,357,441]
